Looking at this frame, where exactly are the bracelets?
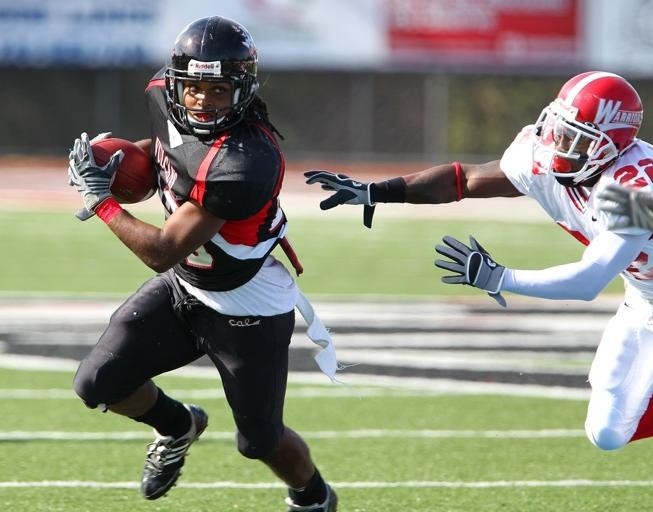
[92,196,125,226]
[369,176,406,204]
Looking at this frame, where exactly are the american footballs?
[91,137,158,202]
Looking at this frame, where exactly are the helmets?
[530,69,644,188]
[163,16,259,138]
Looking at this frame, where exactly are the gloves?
[67,131,126,221]
[304,169,406,229]
[434,233,508,308]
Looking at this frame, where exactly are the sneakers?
[284,484,338,512]
[142,403,208,500]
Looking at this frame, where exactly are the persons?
[66,14,339,512]
[301,69,653,451]
